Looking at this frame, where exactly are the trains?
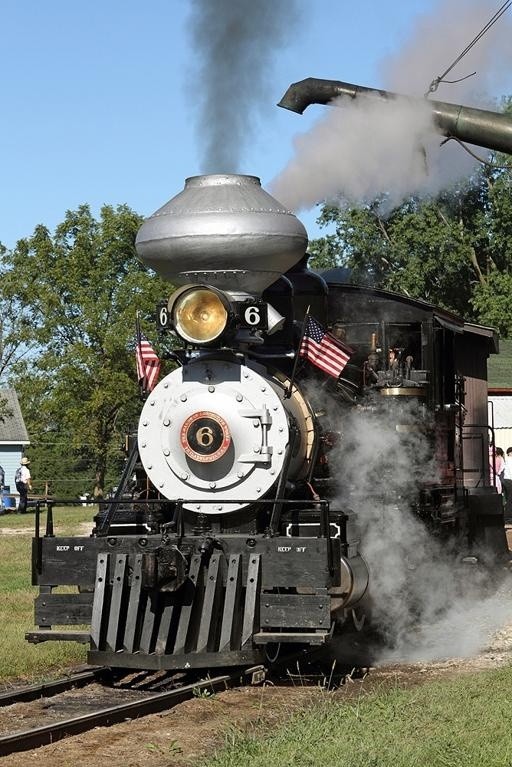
[21,171,504,672]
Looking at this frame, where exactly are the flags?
[137,329,162,393]
[301,318,357,380]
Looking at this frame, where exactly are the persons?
[15,457,34,513]
[487,440,511,526]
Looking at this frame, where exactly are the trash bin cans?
[3,485,11,495]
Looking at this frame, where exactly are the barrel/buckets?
[2,486,10,507]
[5,498,16,508]
